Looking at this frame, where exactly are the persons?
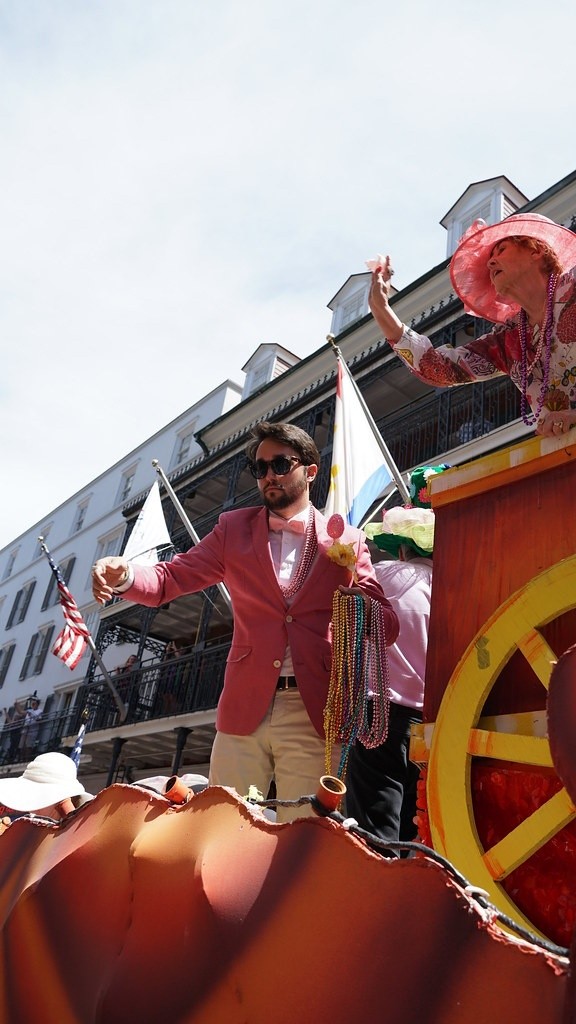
[368,213,576,437]
[344,507,436,859]
[14,697,43,762]
[92,422,400,822]
[117,655,136,674]
[0,752,85,822]
[3,708,23,748]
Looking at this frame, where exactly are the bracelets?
[119,565,128,585]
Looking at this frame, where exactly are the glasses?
[249,456,308,479]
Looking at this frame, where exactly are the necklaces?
[322,589,390,812]
[518,274,559,426]
[280,500,318,599]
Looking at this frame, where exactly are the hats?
[449,212,576,323]
[364,504,436,558]
[0,751,86,812]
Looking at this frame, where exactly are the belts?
[276,676,297,689]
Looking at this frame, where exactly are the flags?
[123,481,172,566]
[45,549,91,671]
[70,725,85,769]
[322,360,394,528]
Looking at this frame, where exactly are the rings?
[554,422,563,428]
[91,571,94,577]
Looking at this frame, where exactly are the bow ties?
[268,516,305,534]
